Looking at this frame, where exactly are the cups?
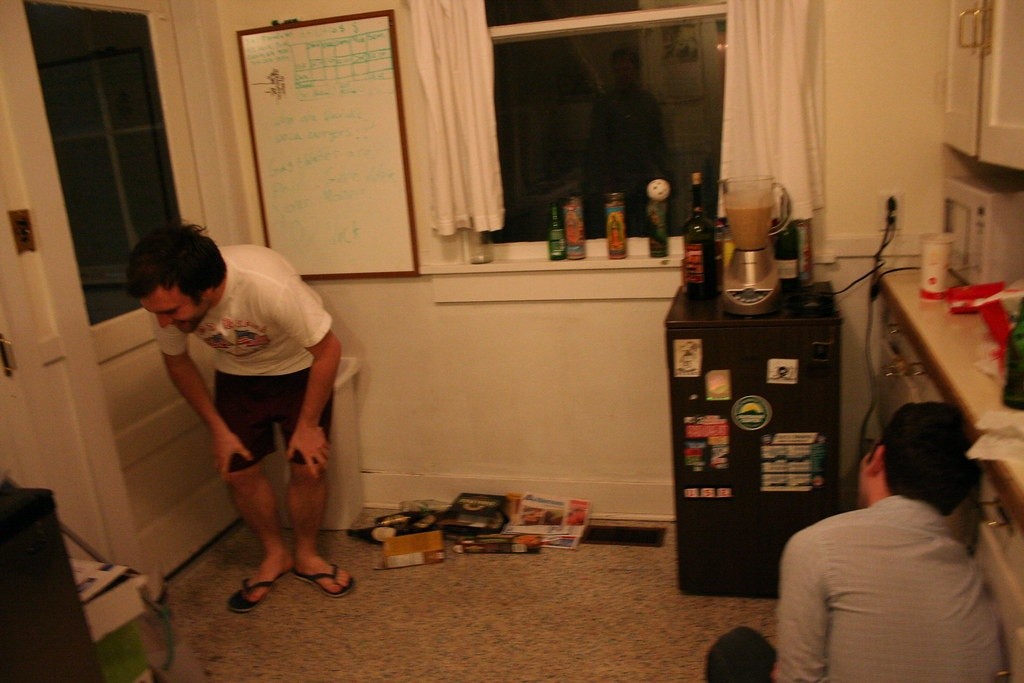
[917,232,955,301]
[468,229,492,264]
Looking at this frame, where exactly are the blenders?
[718,176,790,316]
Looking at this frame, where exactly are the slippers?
[291,564,354,599]
[226,566,294,612]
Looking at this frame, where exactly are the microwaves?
[942,174,1024,286]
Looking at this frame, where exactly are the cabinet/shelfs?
[665,287,844,599]
[878,286,1024,683]
[945,0,1024,169]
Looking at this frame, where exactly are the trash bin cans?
[72,556,157,683]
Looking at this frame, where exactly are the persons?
[591,48,675,225]
[127,224,354,613]
[705,399,1006,682]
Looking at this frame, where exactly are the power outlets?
[873,193,905,230]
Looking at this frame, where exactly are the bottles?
[549,203,566,261]
[347,527,412,544]
[684,173,719,300]
[771,196,800,295]
[375,510,435,527]
[606,191,627,259]
[647,202,668,255]
[396,514,437,536]
[564,197,585,260]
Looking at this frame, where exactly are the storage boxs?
[373,530,446,569]
